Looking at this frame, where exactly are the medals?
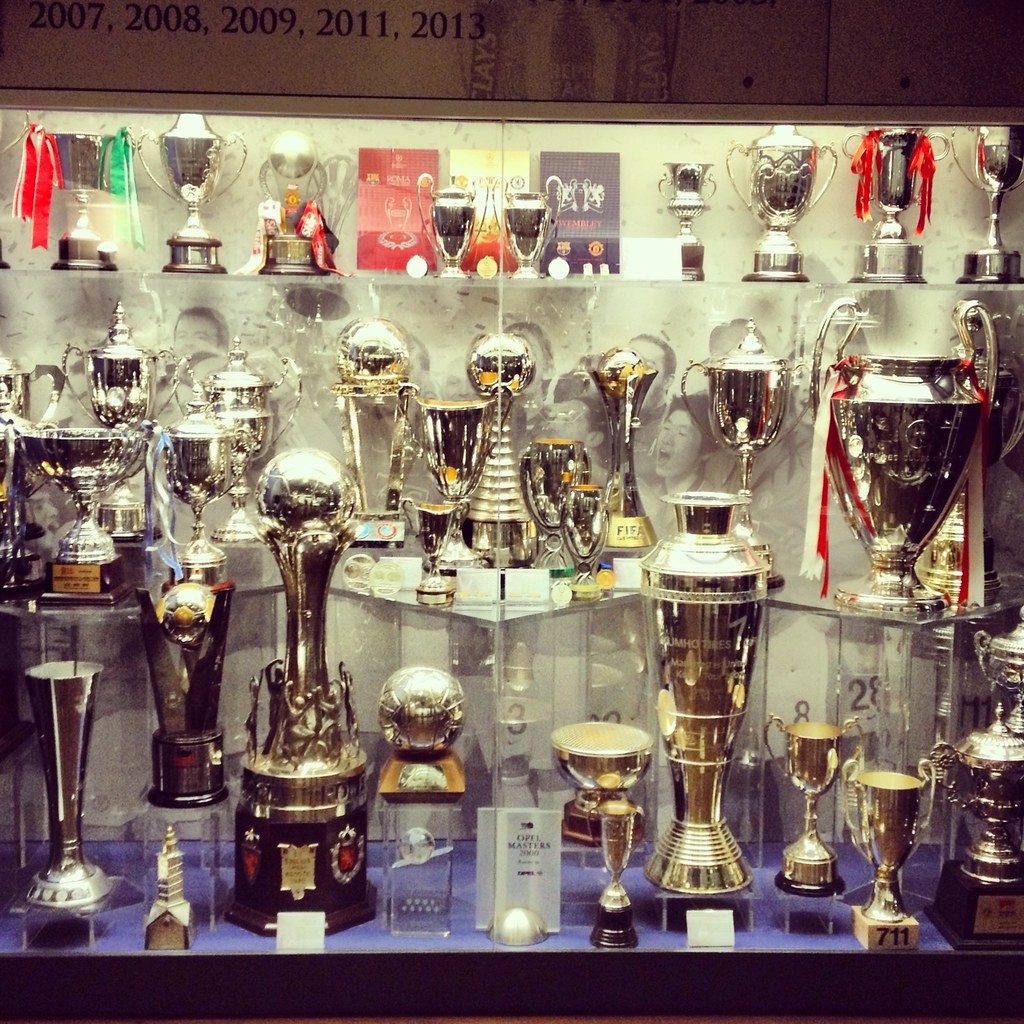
[405,254,429,280]
[476,256,498,280]
[547,257,571,280]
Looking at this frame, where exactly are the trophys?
[0,297,1024,952]
[24,113,1024,283]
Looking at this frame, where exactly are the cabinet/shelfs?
[1,85,1023,1021]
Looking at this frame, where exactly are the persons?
[628,333,675,424]
[785,346,836,426]
[653,394,721,498]
[14,485,63,555]
[708,316,766,356]
[543,396,610,488]
[502,321,552,434]
[159,306,230,380]
